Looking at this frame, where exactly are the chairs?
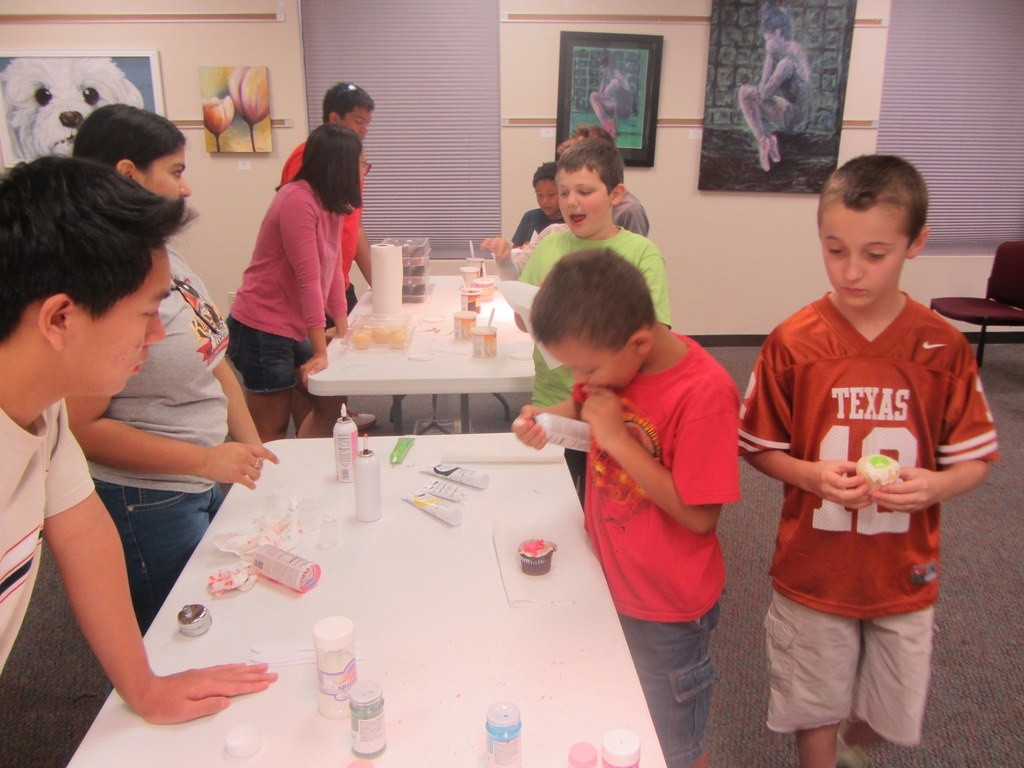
[930,241,1024,367]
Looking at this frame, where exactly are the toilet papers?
[370,242,404,317]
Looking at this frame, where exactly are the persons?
[738,154,1004,768]
[73,104,278,636]
[510,248,742,768]
[223,124,372,438]
[515,138,674,508]
[479,124,649,284]
[0,153,277,725]
[275,82,379,431]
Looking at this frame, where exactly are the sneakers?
[347,410,377,431]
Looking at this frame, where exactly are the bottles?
[333,403,358,484]
[352,433,382,522]
[482,699,521,768]
[568,741,600,768]
[347,680,387,758]
[599,730,641,768]
[512,412,591,452]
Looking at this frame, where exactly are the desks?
[67,434,668,768]
[308,275,536,434]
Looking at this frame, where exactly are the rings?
[254,457,260,468]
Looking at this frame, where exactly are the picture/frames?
[554,30,663,167]
[0,50,165,168]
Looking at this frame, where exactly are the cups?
[313,616,357,720]
[459,288,481,314]
[453,311,477,341]
[474,277,495,303]
[460,266,481,289]
[470,325,498,360]
[466,258,486,278]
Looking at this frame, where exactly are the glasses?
[361,161,371,176]
[346,81,359,92]
